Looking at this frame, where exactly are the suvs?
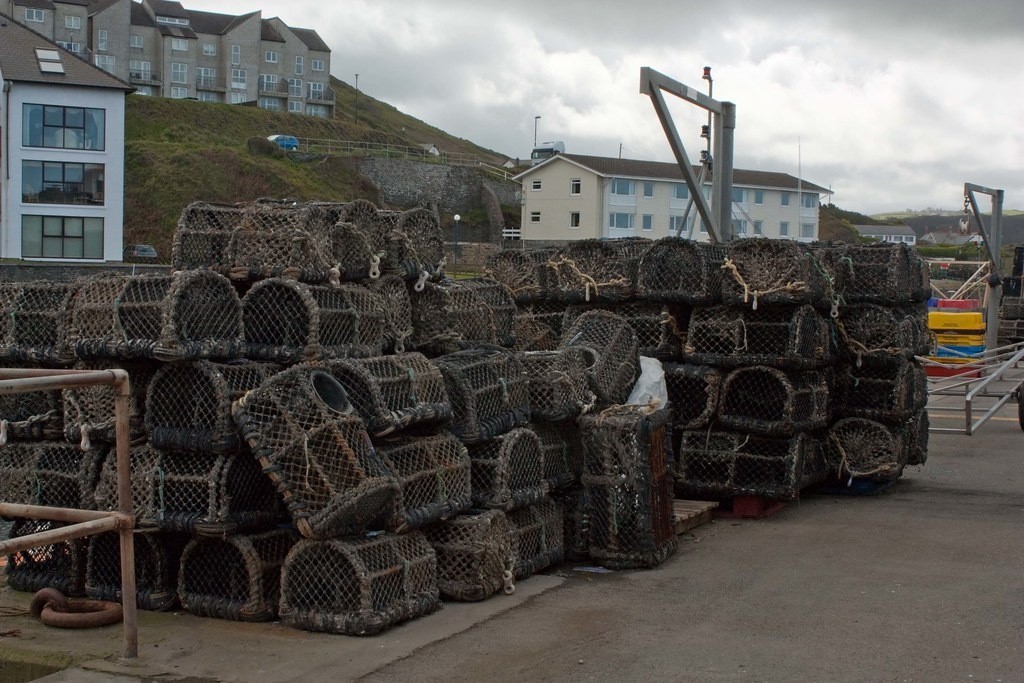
[267,135,299,151]
[125,247,157,257]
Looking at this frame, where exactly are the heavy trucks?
[531,141,565,167]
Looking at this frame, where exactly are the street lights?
[355,73,358,124]
[535,116,541,146]
[454,214,461,279]
